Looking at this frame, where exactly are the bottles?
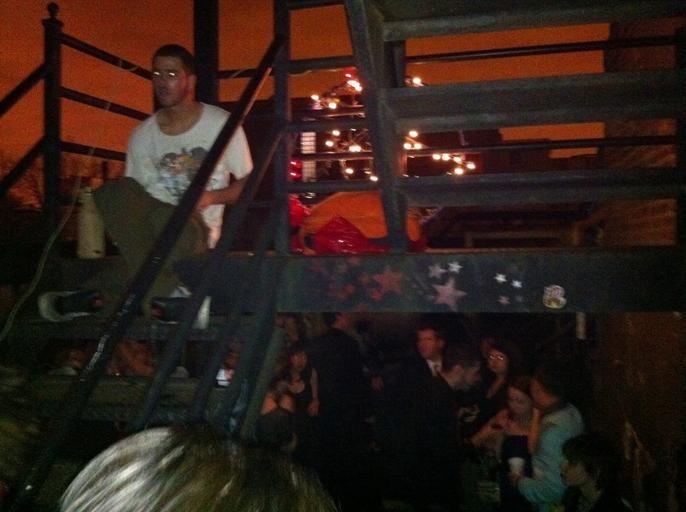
[76,184,107,261]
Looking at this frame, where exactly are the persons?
[0,275,685,511]
[89,40,249,367]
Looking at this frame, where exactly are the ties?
[433,363,443,378]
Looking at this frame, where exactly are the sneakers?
[147,291,208,328]
[37,290,107,323]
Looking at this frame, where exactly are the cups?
[507,456,526,477]
